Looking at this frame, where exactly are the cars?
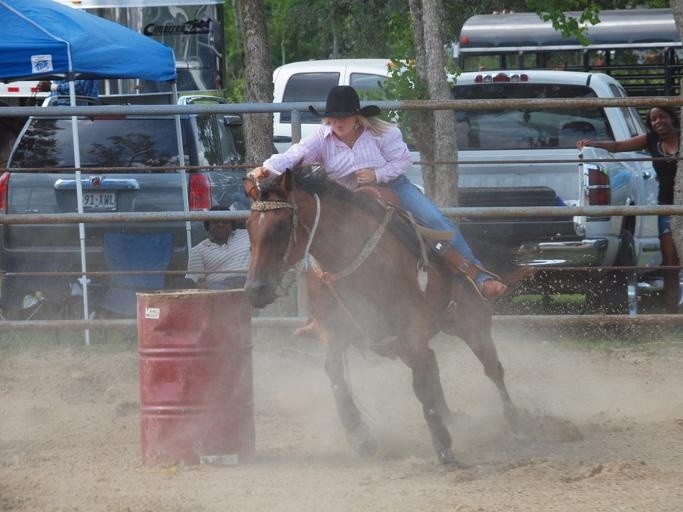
[0,93,279,301]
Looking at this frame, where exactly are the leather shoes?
[483,278,509,303]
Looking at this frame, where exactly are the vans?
[273,57,414,153]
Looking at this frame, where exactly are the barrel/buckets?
[136,288,256,469]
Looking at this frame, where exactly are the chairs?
[0,256,73,348]
[95,232,174,354]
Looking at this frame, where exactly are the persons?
[576,105,682,314]
[180,205,284,316]
[249,86,507,335]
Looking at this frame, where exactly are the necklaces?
[662,134,676,163]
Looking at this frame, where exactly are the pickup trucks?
[408,69,683,314]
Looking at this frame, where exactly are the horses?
[240,157,519,472]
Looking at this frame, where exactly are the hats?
[308,85,381,118]
